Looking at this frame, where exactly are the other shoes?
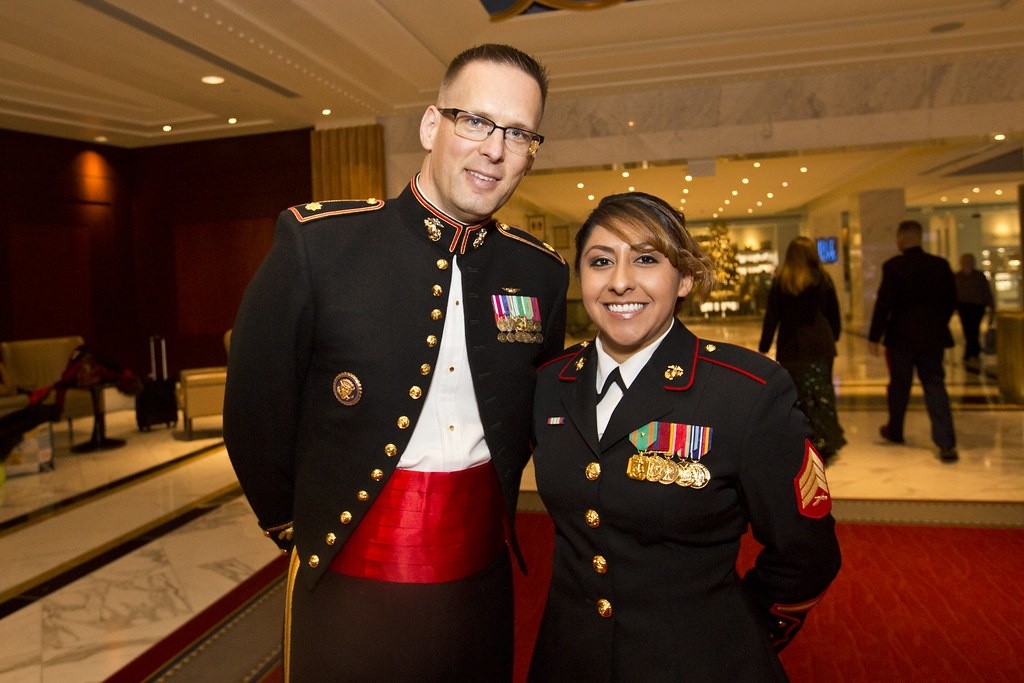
[880,425,905,444]
[940,448,958,461]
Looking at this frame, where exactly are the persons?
[757,235,850,467]
[956,253,997,374]
[869,218,960,462]
[535,192,843,683]
[223,43,571,683]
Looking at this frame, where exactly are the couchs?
[0,395,56,478]
[0,335,94,421]
[179,328,233,442]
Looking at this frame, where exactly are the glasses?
[439,108,545,157]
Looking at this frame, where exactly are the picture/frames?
[553,225,570,250]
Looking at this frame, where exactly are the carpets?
[100,488,1024,683]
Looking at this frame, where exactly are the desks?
[54,379,126,453]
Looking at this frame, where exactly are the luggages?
[135,334,179,432]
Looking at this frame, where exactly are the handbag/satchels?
[985,324,996,355]
